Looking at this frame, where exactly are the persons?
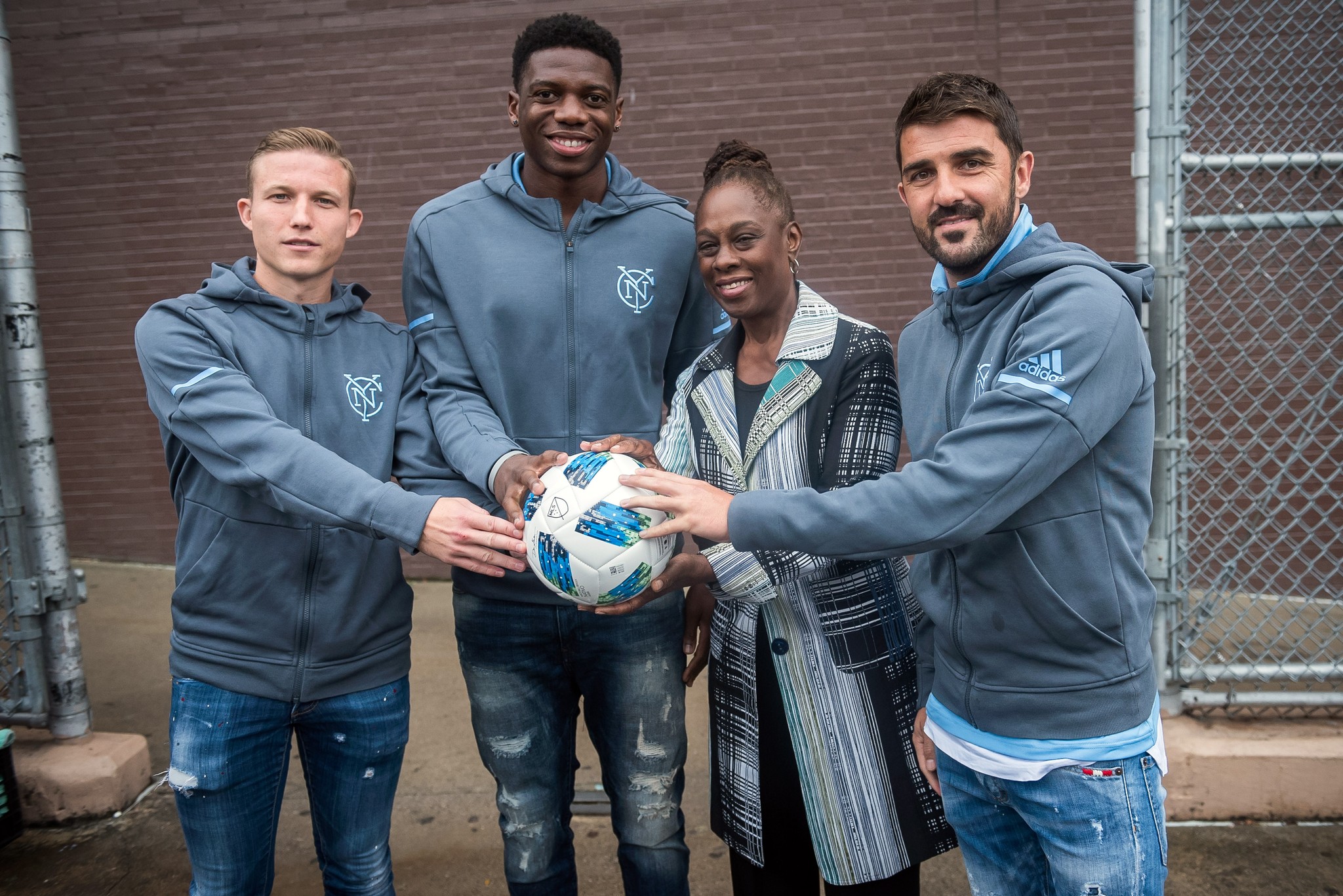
[561,76,1168,896]
[575,125,963,895]
[400,12,719,896]
[139,121,529,896]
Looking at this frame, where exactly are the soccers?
[522,452,677,607]
